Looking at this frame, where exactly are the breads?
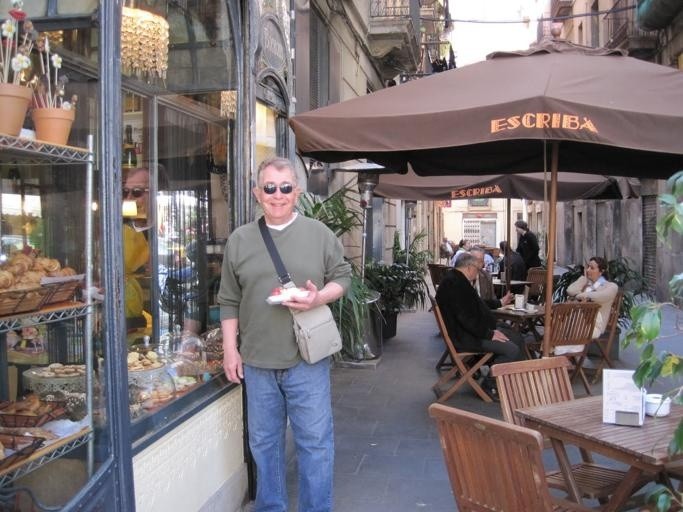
[0,350,173,459]
[0,252,76,292]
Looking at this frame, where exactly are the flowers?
[0,3,77,112]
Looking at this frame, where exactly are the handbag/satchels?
[293,304,343,365]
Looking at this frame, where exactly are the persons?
[434,220,618,407]
[215,155,352,512]
[122,159,206,379]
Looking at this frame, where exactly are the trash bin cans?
[349,289,383,360]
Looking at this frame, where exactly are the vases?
[0,86,75,145]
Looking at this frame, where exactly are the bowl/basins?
[99,362,164,387]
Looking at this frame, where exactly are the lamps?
[120,0,171,84]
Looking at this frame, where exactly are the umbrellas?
[284,28,682,360]
[359,155,640,287]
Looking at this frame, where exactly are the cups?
[526,302,535,310]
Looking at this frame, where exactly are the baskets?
[0,433,47,471]
[0,400,67,427]
[1,272,86,317]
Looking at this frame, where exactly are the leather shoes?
[481,378,500,402]
[456,365,481,380]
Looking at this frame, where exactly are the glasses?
[122,187,150,199]
[258,183,298,194]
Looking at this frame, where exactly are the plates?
[525,310,539,314]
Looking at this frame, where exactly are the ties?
[473,279,477,292]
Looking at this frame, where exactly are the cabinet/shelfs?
[0,137,95,512]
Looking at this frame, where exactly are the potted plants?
[297,189,433,359]
